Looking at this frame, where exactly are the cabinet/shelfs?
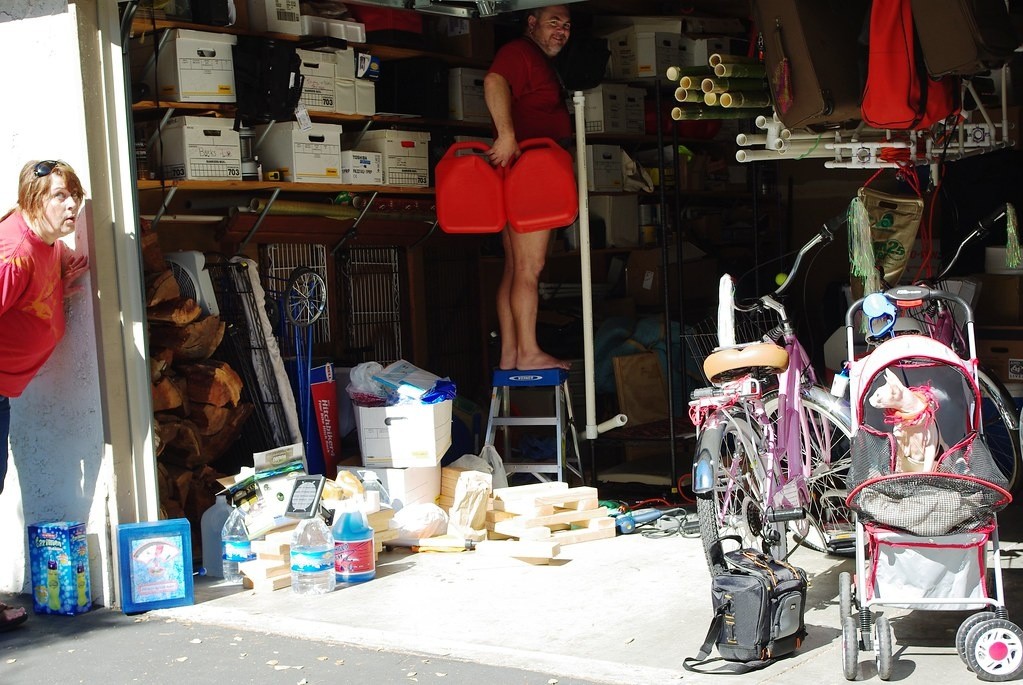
[126,19,447,192]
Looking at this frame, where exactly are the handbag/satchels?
[683,534,808,675]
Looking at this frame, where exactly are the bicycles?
[687,206,1010,580]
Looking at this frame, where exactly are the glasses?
[34,160,56,177]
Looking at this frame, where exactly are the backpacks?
[862,0,964,130]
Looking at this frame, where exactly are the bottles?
[507,138,580,233]
[436,141,507,232]
[220,507,255,584]
[331,508,376,582]
[201,495,234,577]
[290,516,335,594]
[361,471,391,506]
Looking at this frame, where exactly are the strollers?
[837,286,1023,681]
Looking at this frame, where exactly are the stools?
[479,367,587,488]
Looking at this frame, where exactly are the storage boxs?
[117,518,194,613]
[253,120,342,184]
[246,0,366,44]
[296,46,376,116]
[214,398,453,542]
[340,150,383,186]
[154,117,243,181]
[127,28,236,104]
[979,383,1023,475]
[971,325,1023,384]
[356,129,430,187]
[27,520,92,617]
[561,20,738,311]
[429,12,495,123]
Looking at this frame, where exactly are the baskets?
[908,279,957,335]
[682,304,775,386]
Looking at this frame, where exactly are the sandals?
[0,604,28,629]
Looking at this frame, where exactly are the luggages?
[757,0,867,134]
[912,0,1013,80]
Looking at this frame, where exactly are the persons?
[0,160,89,495]
[483,5,575,370]
